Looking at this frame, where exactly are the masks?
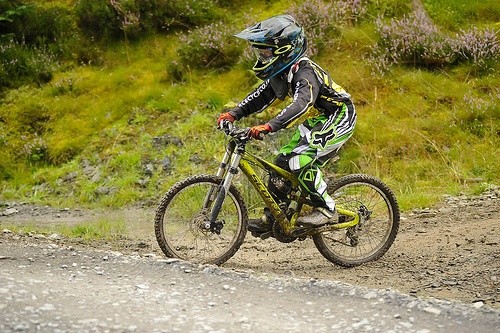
[257,54,278,66]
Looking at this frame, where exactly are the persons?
[216,15,358,235]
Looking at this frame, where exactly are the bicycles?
[154,119,401,270]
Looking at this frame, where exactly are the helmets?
[232,14,308,81]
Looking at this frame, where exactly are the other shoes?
[247,214,275,231]
[296,206,339,226]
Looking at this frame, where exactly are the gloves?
[250,124,272,140]
[216,112,235,127]
